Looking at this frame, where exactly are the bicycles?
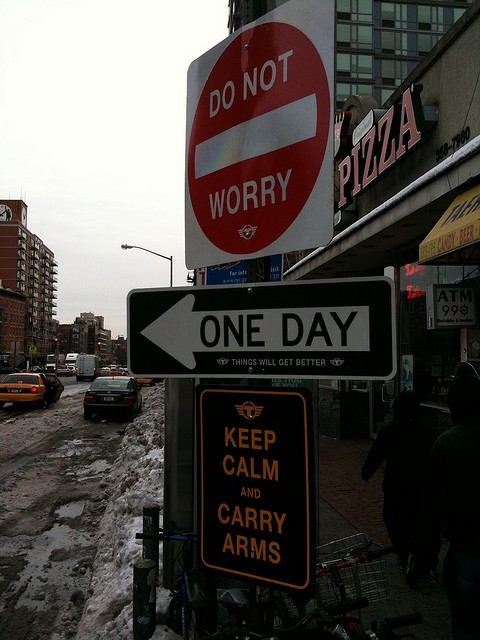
[136,532,422,640]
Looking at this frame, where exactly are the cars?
[101,368,109,374]
[0,372,64,410]
[136,377,154,385]
[120,368,128,372]
[84,376,141,421]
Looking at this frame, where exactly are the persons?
[430,362,479,631]
[360,392,428,576]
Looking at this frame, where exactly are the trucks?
[65,353,78,374]
[46,354,65,372]
[77,354,100,382]
[110,365,116,370]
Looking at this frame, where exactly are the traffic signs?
[190,383,318,591]
[127,276,401,381]
[185,0,337,266]
